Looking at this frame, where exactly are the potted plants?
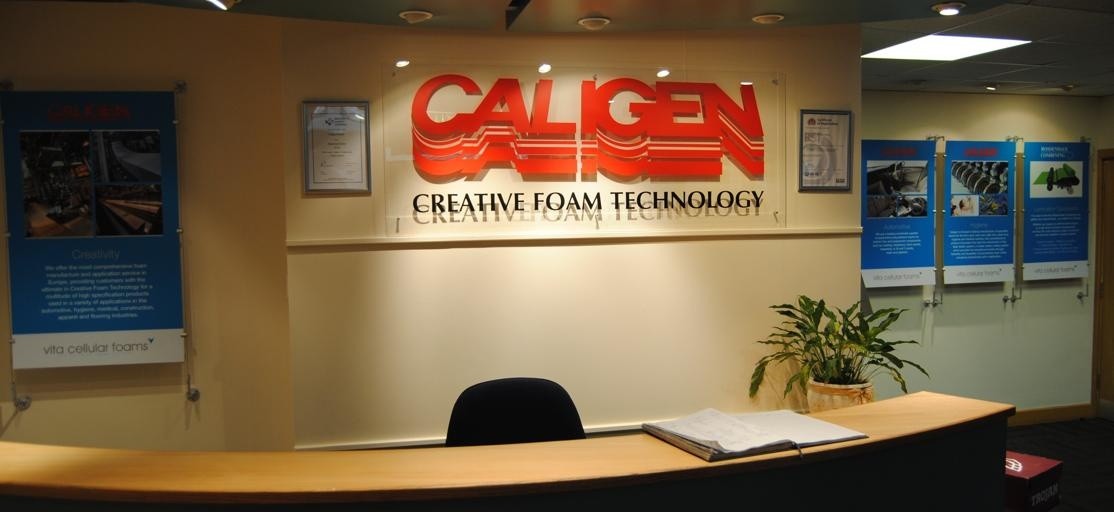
[747,293,930,413]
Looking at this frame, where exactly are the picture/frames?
[798,109,852,192]
[303,99,373,198]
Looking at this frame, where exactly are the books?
[641,407,868,464]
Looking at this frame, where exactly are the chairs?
[448,376,586,447]
